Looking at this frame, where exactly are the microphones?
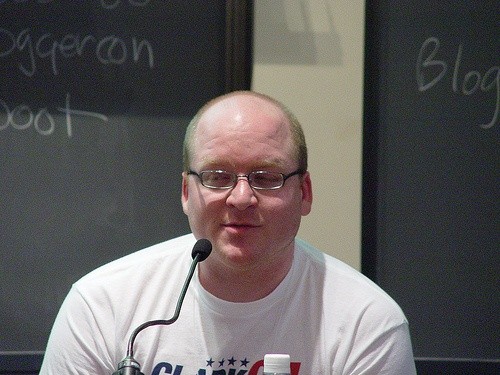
[111,239,213,375]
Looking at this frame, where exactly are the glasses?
[184,168,306,190]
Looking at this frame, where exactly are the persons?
[35,88,418,374]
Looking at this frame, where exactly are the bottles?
[263,353,291,375]
